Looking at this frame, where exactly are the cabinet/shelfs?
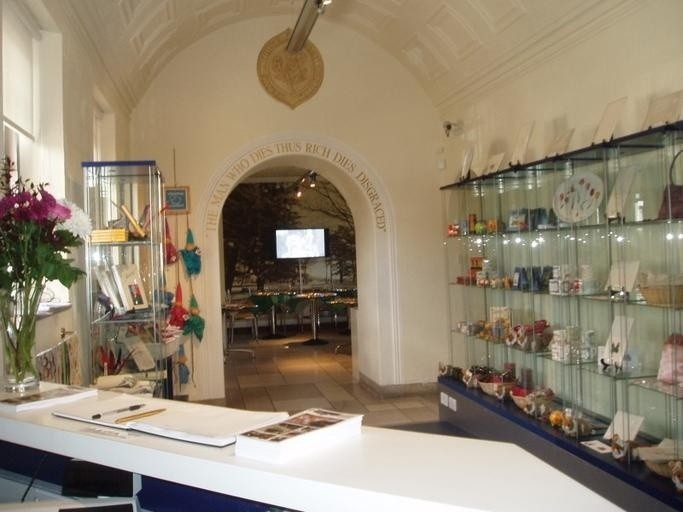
[436,119,683,511]
[80,159,170,400]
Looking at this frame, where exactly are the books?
[53,395,290,447]
[235,407,363,447]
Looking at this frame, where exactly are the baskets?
[642,459,683,478]
[478,379,517,396]
[638,283,682,308]
[509,390,555,411]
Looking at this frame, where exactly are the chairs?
[224,288,360,344]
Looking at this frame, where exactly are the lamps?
[294,170,317,198]
[285,1,331,54]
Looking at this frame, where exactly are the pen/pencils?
[92,404,167,424]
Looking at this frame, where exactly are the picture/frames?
[94,260,148,312]
[123,334,156,372]
[162,183,192,216]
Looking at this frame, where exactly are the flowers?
[0,154,93,385]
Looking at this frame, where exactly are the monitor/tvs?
[274,227,330,260]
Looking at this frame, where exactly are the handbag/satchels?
[658,183,682,218]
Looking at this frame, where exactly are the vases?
[0,286,47,406]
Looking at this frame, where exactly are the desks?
[0,377,625,512]
